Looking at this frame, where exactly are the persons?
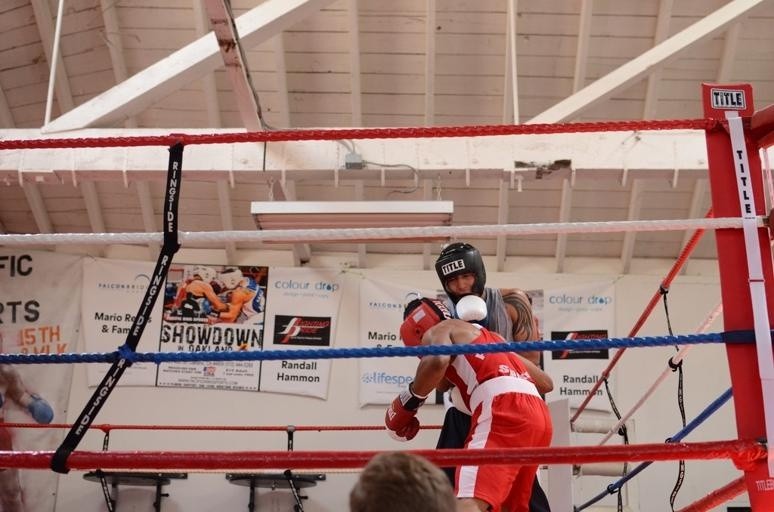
[433,240,551,512]
[201,265,264,326]
[0,335,56,511]
[164,264,231,325]
[384,295,555,512]
[347,450,458,511]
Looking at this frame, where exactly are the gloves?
[452,295,490,327]
[384,405,420,442]
[27,393,53,424]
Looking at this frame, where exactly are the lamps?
[249,199,455,242]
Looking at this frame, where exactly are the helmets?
[193,266,243,290]
[401,297,453,359]
[435,242,486,302]
